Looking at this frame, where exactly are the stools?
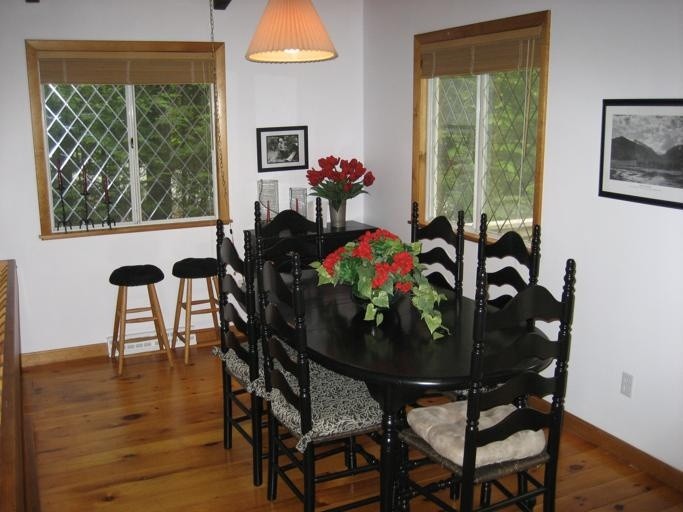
[171,256,220,364]
[108,262,174,377]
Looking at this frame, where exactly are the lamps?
[243,1,340,65]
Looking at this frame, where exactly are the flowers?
[304,153,377,215]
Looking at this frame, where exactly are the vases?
[328,199,347,230]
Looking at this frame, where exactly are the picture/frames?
[596,98,683,211]
[254,125,309,174]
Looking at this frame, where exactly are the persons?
[266,136,298,162]
